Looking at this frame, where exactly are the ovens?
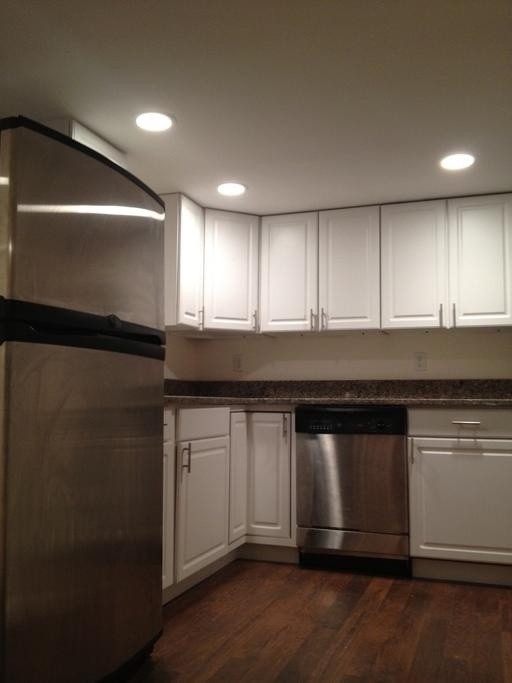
[294,401,412,568]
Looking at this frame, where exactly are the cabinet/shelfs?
[261,204,382,337]
[246,409,297,553]
[163,405,175,590]
[381,192,512,333]
[158,192,204,337]
[175,406,230,585]
[204,206,262,341]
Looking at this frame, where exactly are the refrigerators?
[1,114,173,682]
